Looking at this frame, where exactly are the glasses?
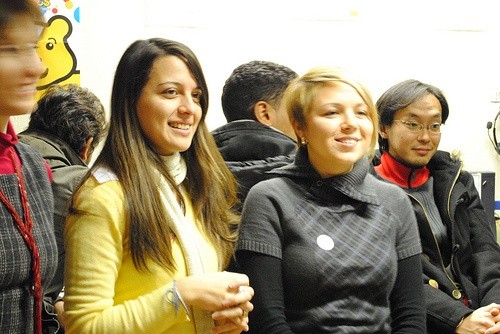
[392,120,440,134]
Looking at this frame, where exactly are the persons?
[64,38,254,334]
[210,60,298,232]
[0,0,58,334]
[369,78,500,334]
[16,84,105,334]
[235,67,426,334]
